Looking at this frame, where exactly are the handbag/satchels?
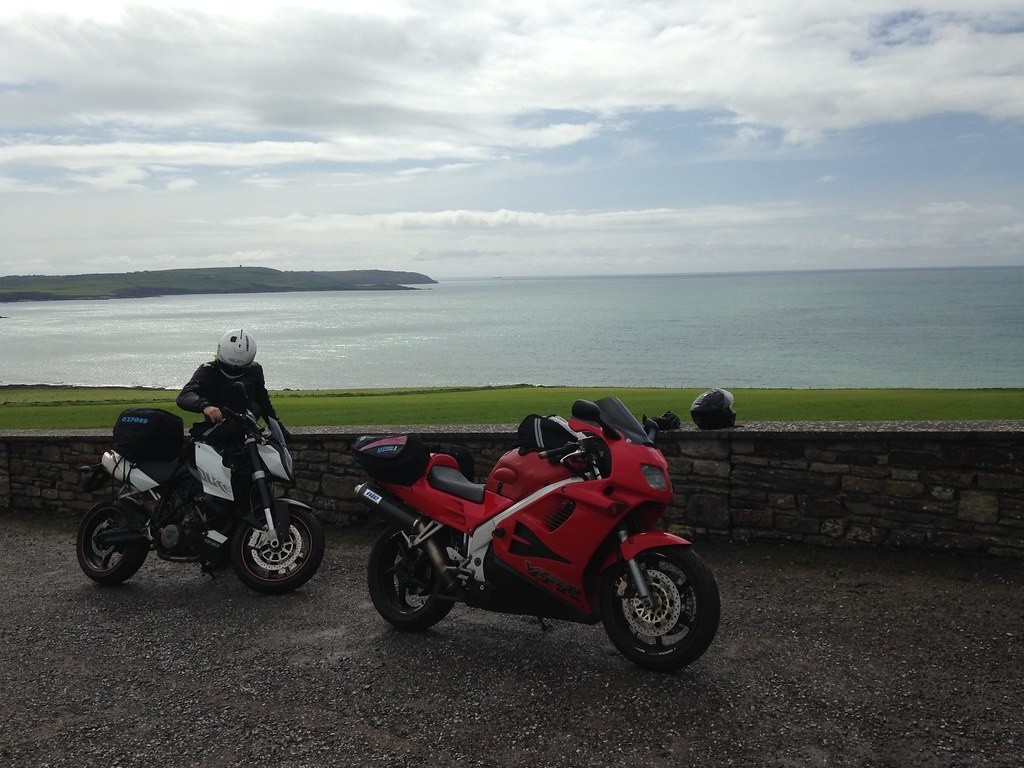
[352,433,430,486]
[425,443,475,483]
[113,407,184,459]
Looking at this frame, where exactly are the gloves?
[643,412,681,439]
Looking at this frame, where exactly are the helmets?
[691,388,736,429]
[216,328,257,380]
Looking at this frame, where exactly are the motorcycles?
[350,396,723,673]
[76,381,326,595]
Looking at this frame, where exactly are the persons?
[176,329,293,576]
